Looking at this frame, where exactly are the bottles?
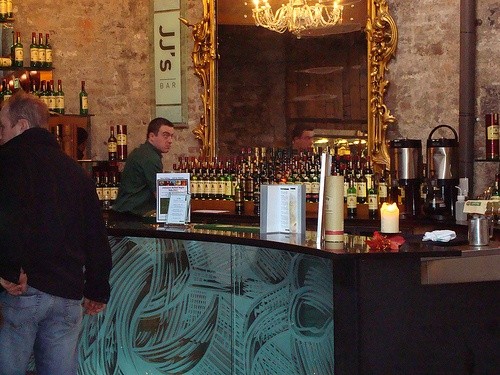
[107,126,117,161]
[368,180,379,223]
[10,31,53,69]
[235,182,245,215]
[254,184,261,216]
[79,80,89,115]
[0,78,64,115]
[173,145,392,207]
[346,177,356,221]
[95,174,119,208]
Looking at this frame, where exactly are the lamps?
[251,0,343,39]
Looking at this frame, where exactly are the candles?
[380,201,399,233]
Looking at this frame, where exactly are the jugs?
[467,214,494,245]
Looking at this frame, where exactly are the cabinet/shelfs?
[43,113,96,178]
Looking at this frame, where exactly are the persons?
[279,124,315,157]
[0,90,111,374]
[114,117,174,217]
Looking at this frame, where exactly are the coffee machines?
[390,124,458,221]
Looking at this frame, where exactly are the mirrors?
[178,0,396,175]
